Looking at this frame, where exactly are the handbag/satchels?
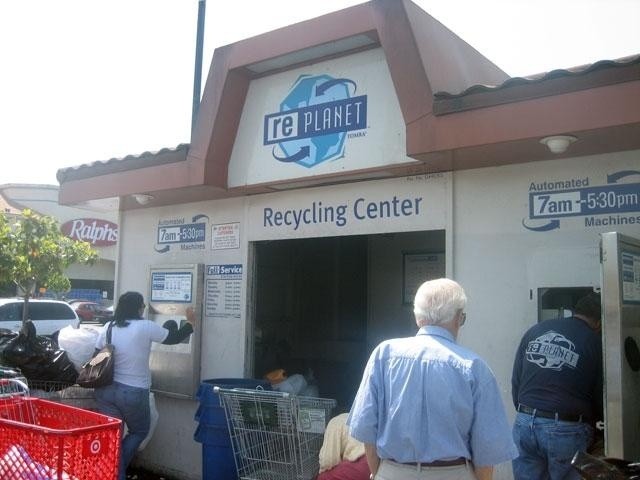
[74,342,116,389]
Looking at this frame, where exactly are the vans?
[0,297,81,337]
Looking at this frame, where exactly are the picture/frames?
[400,250,446,306]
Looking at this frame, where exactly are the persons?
[93,290,197,480]
[510,292,604,480]
[344,278,521,480]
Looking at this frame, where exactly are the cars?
[67,299,113,324]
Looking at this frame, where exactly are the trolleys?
[0,375,124,480]
[213,386,338,479]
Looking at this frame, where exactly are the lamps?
[539,136,578,154]
[132,194,154,205]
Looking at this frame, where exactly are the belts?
[515,403,590,426]
[385,455,469,467]
